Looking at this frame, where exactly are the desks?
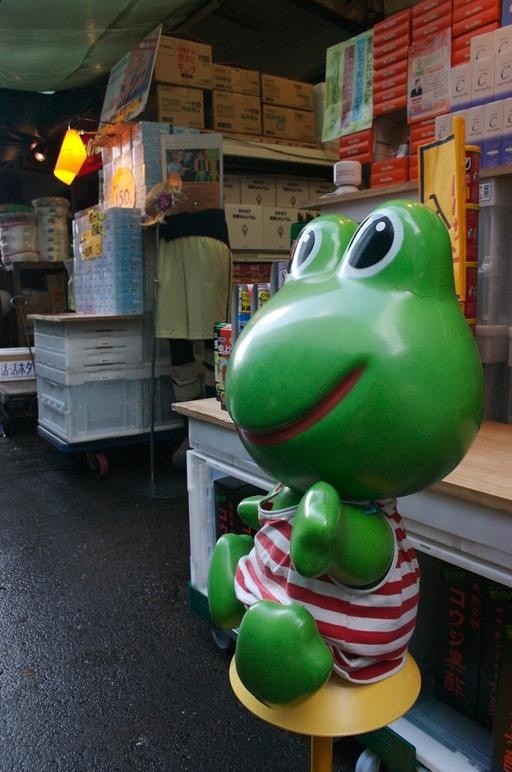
[168,386,512,770]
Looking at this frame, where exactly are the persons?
[153,182,234,477]
[408,77,423,97]
[168,150,190,181]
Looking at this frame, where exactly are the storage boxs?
[151,34,335,261]
[336,3,511,195]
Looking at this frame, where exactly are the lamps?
[32,114,115,186]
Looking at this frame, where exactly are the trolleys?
[36,420,188,482]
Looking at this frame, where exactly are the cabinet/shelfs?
[28,310,151,450]
[0,137,73,350]
[151,138,337,432]
